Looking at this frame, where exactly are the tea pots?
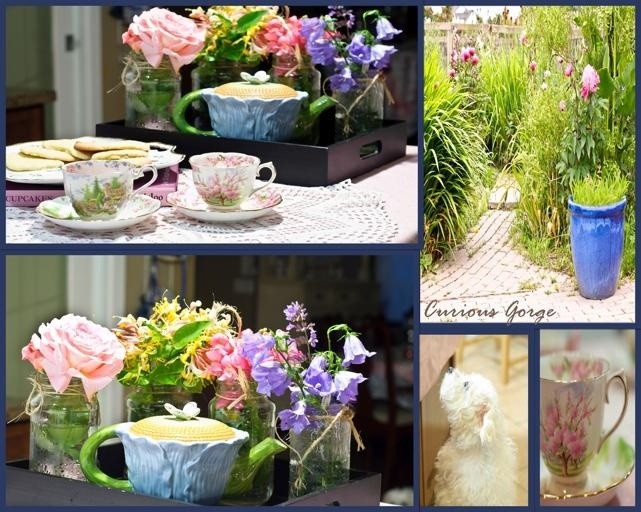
[171,66,338,140]
[80,402,291,502]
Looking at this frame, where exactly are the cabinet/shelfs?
[6,91,56,146]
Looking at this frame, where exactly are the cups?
[535,352,628,482]
[60,158,158,221]
[186,151,277,206]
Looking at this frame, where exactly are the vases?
[288,404,351,502]
[26,374,100,483]
[269,56,322,146]
[207,381,276,506]
[122,63,181,131]
[337,71,385,156]
[191,60,260,135]
[122,384,192,424]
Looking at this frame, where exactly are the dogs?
[430,365,522,507]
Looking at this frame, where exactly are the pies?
[6,136,151,172]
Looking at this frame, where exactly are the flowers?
[196,327,303,454]
[121,6,207,122]
[236,302,378,436]
[111,288,232,422]
[22,314,125,463]
[300,5,403,124]
[250,13,341,84]
[185,6,278,88]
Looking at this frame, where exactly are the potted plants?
[567,155,631,301]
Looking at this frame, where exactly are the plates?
[4,135,185,185]
[168,184,284,224]
[35,196,163,230]
[529,431,637,501]
